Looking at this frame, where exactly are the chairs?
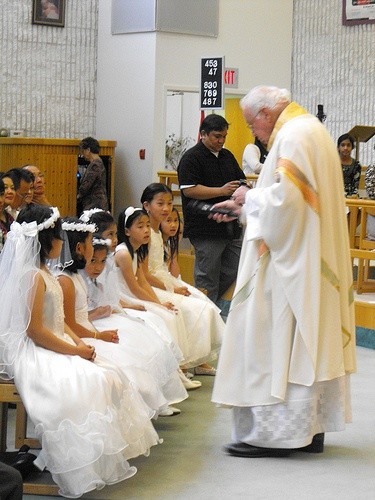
[345,166,375,294]
[0,376,63,497]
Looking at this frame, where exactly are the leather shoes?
[292,432,325,453]
[224,442,292,458]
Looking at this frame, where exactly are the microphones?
[188,200,238,216]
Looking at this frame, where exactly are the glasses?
[246,109,265,129]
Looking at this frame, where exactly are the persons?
[76,137,109,212]
[38,181,225,421]
[176,114,253,305]
[41,0,60,19]
[0,163,52,250]
[336,133,362,231]
[365,142,375,240]
[1,204,161,499]
[242,136,268,188]
[208,84,357,458]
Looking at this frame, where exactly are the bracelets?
[93,331,98,339]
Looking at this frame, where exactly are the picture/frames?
[32,0,65,27]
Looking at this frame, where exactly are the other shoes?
[168,405,181,414]
[194,366,217,375]
[182,380,202,390]
[156,409,173,416]
[182,370,194,379]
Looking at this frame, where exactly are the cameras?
[239,179,247,186]
